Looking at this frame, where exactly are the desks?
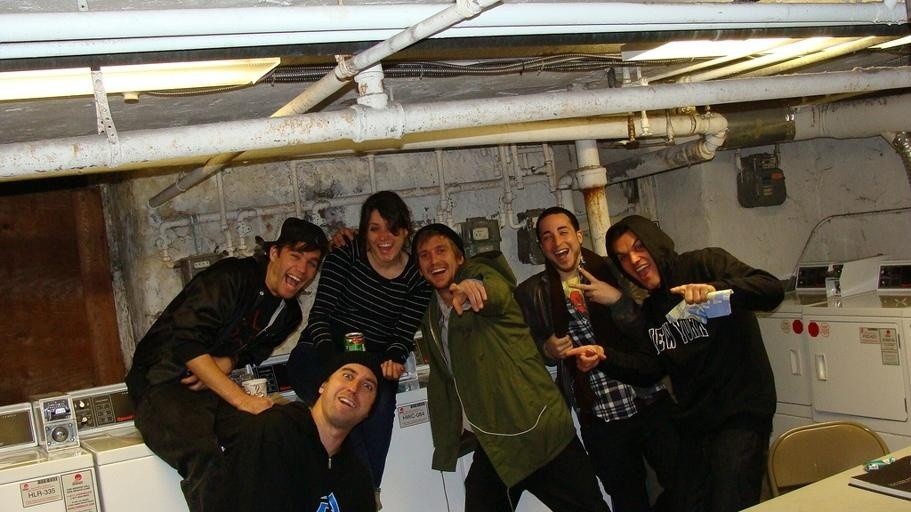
[736,439,910,512]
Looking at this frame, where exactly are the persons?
[291,190,436,511]
[205,350,386,512]
[518,206,703,509]
[563,215,784,511]
[412,221,608,511]
[123,216,330,512]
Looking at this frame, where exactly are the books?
[848,455,911,501]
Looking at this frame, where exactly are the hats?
[254,218,325,248]
[411,223,462,267]
[316,351,380,388]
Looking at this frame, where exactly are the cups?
[242,378,268,397]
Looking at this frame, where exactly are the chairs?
[767,420,890,496]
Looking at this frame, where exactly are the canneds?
[343,332,366,352]
